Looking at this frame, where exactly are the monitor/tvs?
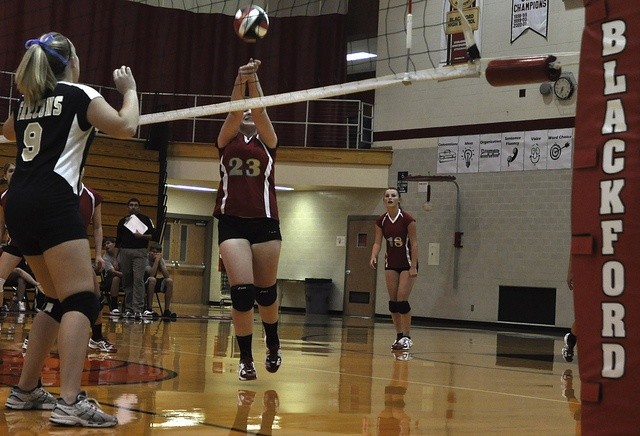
[497,285,555,326]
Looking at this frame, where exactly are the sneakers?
[163,318,177,322]
[144,319,154,323]
[19,300,26,311]
[390,338,413,348]
[163,310,177,317]
[88,337,118,352]
[238,361,257,381]
[18,313,26,323]
[134,312,142,319]
[562,331,576,360]
[264,335,282,373]
[147,307,159,316]
[48,390,119,428]
[392,336,409,349]
[110,308,120,315]
[5,376,57,410]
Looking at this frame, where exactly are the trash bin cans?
[305,279,331,313]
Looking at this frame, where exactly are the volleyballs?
[234,5,269,43]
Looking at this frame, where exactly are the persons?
[103,236,124,323]
[0,161,14,247]
[4,32,138,429]
[561,236,576,362]
[74,162,117,353]
[370,189,418,350]
[213,58,282,381]
[144,244,177,322]
[114,199,155,321]
